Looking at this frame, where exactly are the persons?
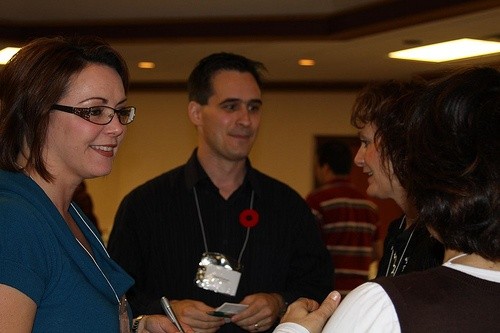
[273,63,500,333]
[0,36,136,333]
[305,140,386,301]
[107,51,335,333]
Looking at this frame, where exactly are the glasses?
[51,104,137,125]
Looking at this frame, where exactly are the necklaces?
[445,253,467,264]
[69,204,121,305]
[385,212,420,277]
[16,162,34,181]
[192,188,253,271]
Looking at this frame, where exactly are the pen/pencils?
[206,310,238,318]
[161,296,185,333]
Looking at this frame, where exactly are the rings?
[254,323,259,331]
